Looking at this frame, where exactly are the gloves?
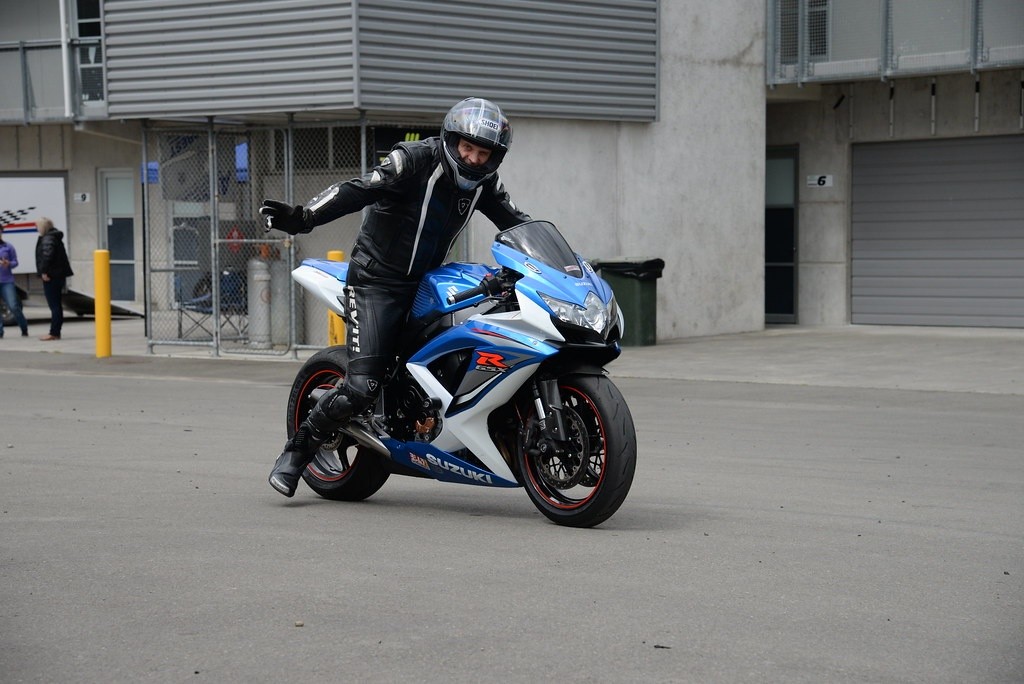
[260,199,303,235]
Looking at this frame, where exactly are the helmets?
[440,96,511,191]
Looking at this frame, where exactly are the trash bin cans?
[591,257,666,347]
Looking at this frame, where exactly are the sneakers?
[268,438,312,498]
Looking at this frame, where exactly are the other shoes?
[22,331,27,335]
[40,334,60,340]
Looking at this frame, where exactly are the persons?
[33,217,75,342]
[0,223,31,339]
[258,96,534,497]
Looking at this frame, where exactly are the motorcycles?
[288,219,638,528]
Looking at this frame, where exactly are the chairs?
[179,265,248,346]
